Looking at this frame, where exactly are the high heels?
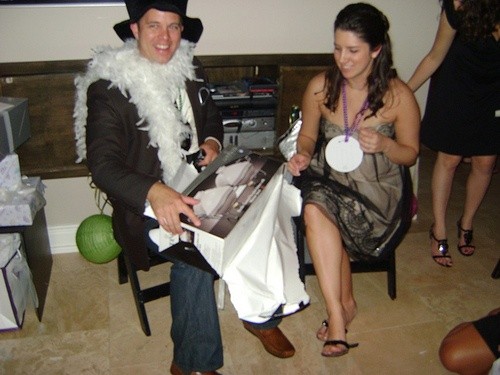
[457,216,475,256]
[429,221,455,267]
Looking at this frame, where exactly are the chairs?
[112,209,177,337]
[275,66,399,303]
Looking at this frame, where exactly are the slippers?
[317,308,361,358]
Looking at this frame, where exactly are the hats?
[113,0,204,44]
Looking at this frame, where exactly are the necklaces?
[325,78,370,173]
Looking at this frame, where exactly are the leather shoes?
[171,356,220,375]
[242,319,296,358]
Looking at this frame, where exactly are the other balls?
[75,214,122,265]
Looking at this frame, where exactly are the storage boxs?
[0,95,57,330]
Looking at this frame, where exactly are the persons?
[287,2,421,358]
[407,0,500,267]
[437,306,500,375]
[86,0,296,375]
